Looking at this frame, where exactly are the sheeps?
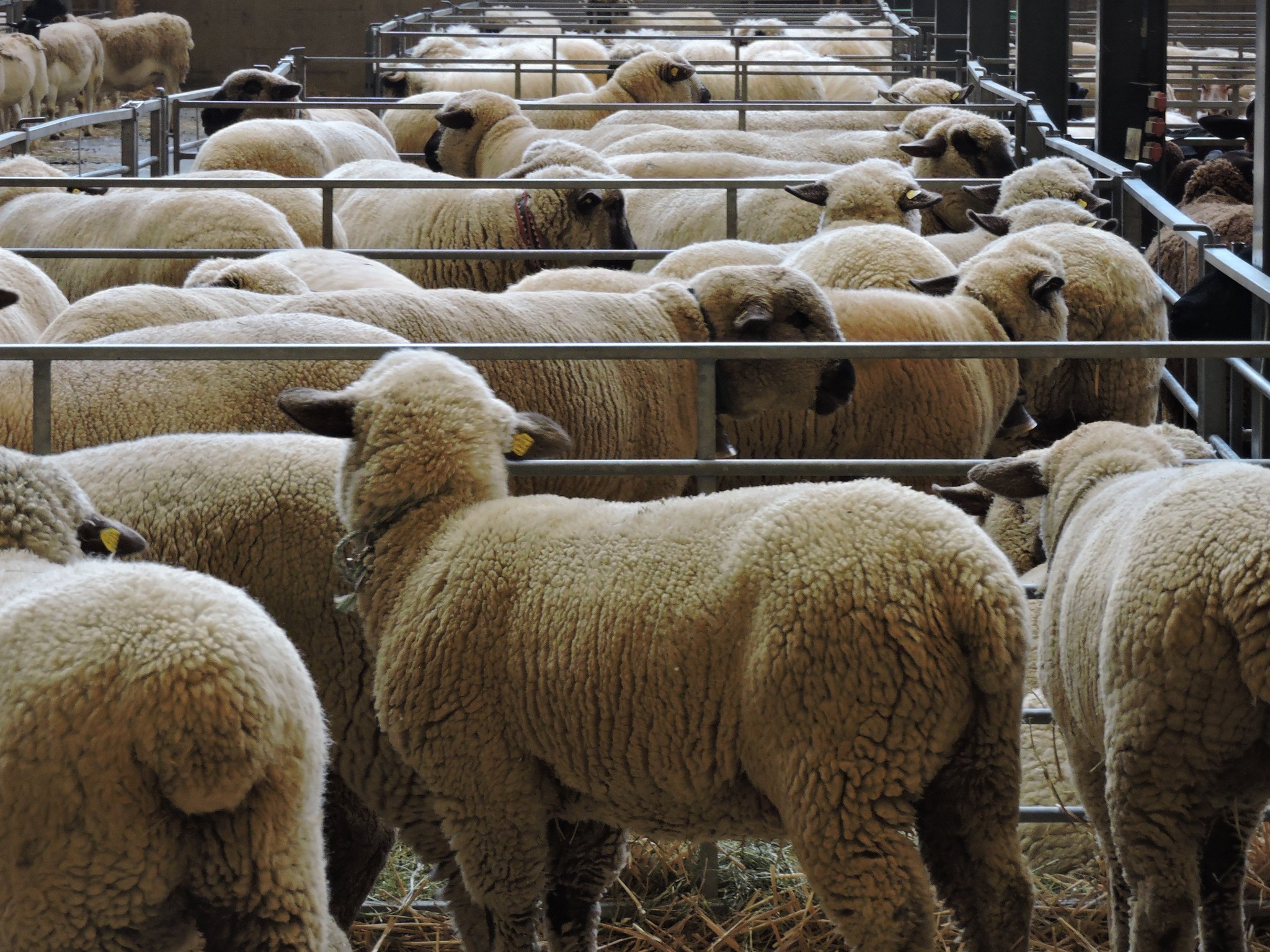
[1,2,1269,950]
